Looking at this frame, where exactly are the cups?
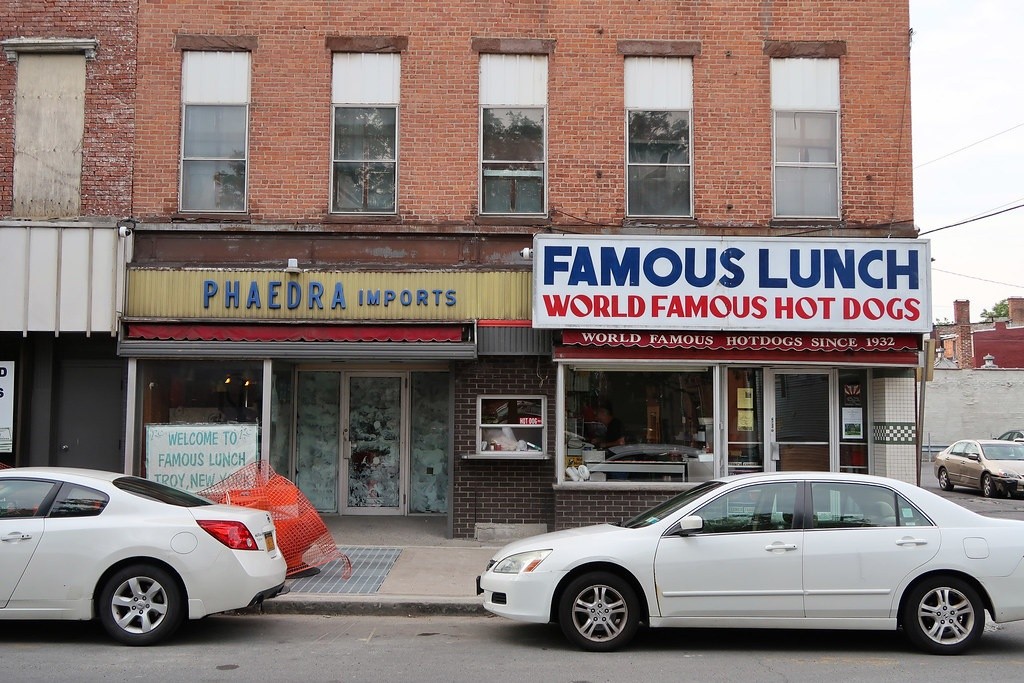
[496,444,501,450]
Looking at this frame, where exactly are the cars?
[476,470,1024,655]
[598,443,711,482]
[933,439,1024,499]
[0,466,291,647]
[991,430,1024,444]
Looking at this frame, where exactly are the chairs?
[741,502,819,531]
[860,502,902,527]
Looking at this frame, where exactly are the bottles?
[490,442,495,451]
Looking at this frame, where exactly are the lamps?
[286,259,301,274]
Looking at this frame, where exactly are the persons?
[591,406,626,458]
